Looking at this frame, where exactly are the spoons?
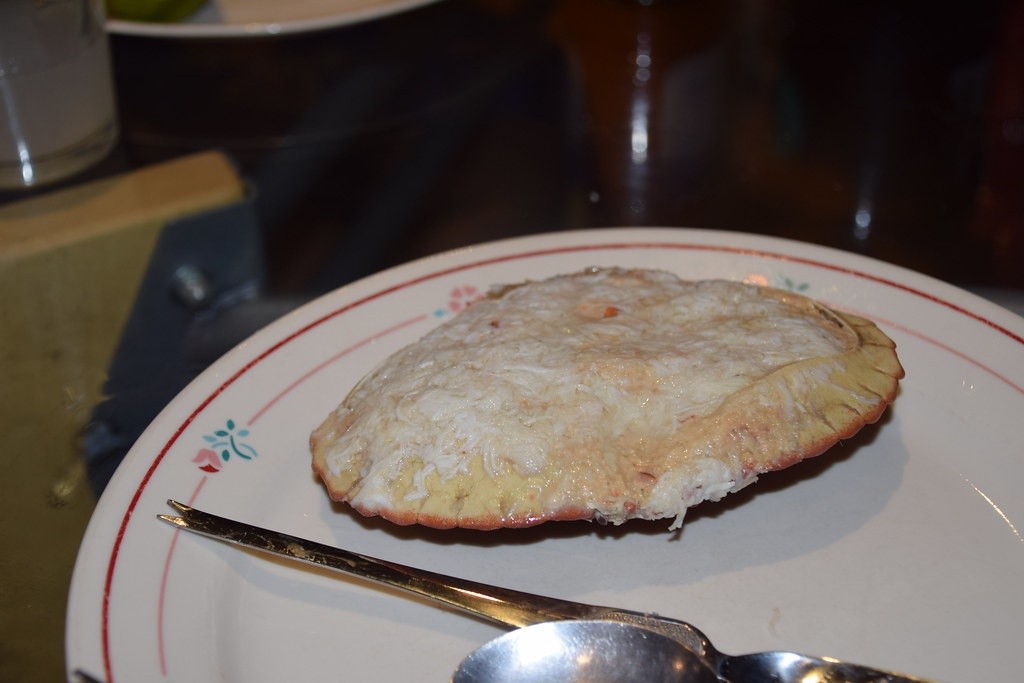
[452,620,721,683]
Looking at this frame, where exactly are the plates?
[105,0,441,38]
[70,229,1024,683]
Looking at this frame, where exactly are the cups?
[0,0,120,188]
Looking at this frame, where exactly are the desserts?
[306,267,904,530]
